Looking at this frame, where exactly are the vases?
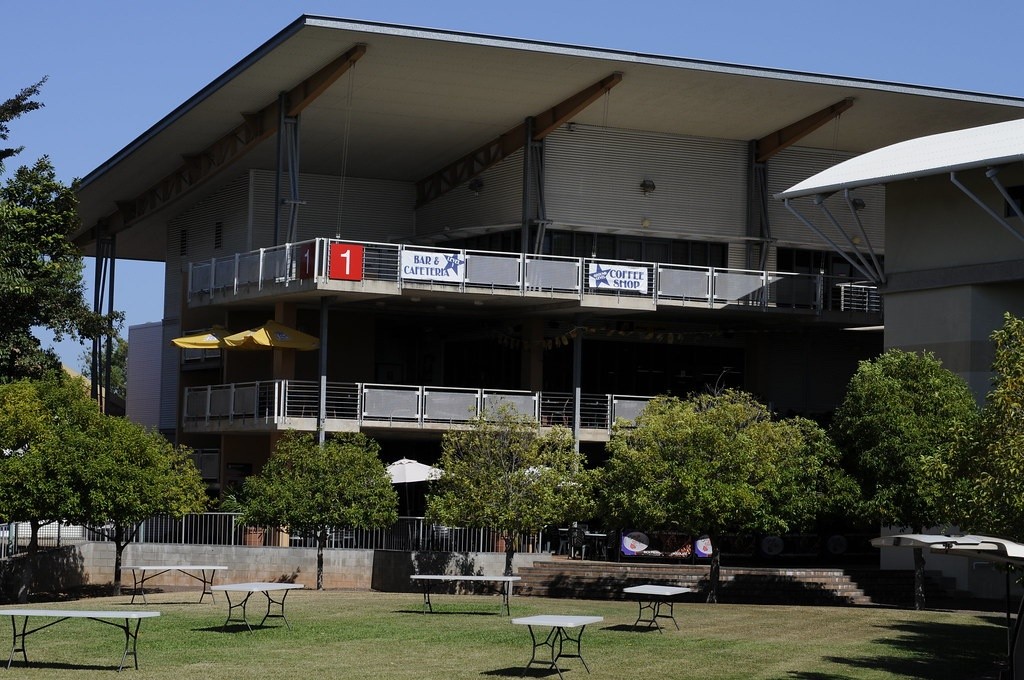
[492,529,516,552]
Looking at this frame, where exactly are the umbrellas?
[168,325,236,383]
[223,320,320,380]
[501,464,584,490]
[386,457,455,516]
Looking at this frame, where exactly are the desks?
[119,566,228,606]
[210,582,304,635]
[510,615,603,680]
[410,575,521,619]
[0,610,160,675]
[624,585,694,635]
[585,403,608,427]
[558,528,607,560]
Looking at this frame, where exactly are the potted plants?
[216,484,265,546]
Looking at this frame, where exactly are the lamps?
[852,235,862,246]
[641,220,649,227]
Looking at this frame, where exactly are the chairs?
[567,527,593,561]
[550,398,570,428]
[598,530,620,562]
